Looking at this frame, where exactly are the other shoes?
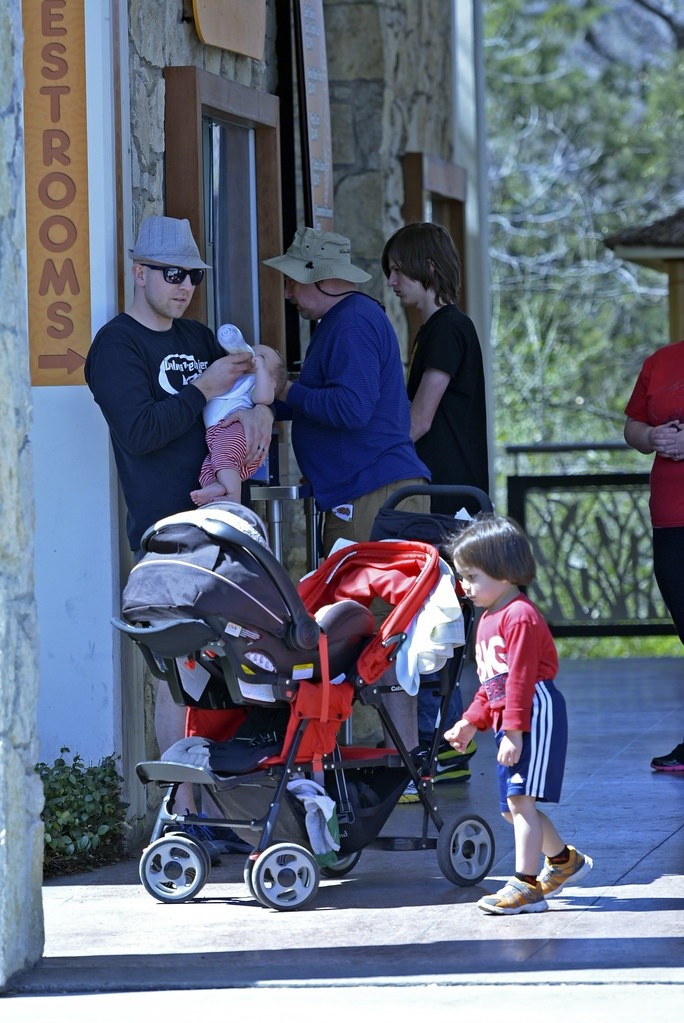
[396,779,425,803]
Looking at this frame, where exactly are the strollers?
[107,480,494,913]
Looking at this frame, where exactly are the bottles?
[217,324,255,361]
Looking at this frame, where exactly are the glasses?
[138,263,205,287]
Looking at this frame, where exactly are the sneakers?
[477,877,549,915]
[535,845,593,899]
[651,744,684,771]
[163,812,254,865]
[406,740,478,787]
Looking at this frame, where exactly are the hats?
[127,214,212,270]
[263,228,372,283]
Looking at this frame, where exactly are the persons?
[190,345,288,506]
[443,514,594,914]
[261,229,431,803]
[86,218,281,867]
[382,222,490,785]
[625,340,684,770]
